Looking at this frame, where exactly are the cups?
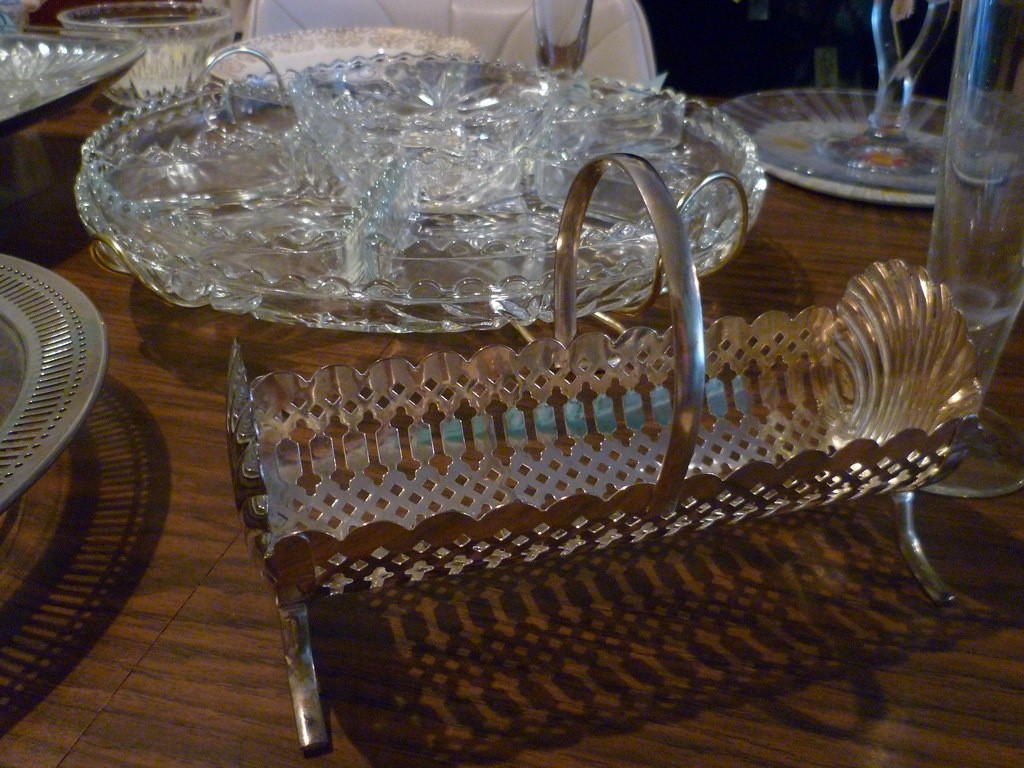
[829,0,1024,497]
[813,0,953,179]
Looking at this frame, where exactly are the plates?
[202,27,474,102]
[706,85,1024,208]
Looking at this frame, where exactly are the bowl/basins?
[287,49,554,215]
[56,1,235,111]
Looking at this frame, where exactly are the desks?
[0,47,1024,768]
